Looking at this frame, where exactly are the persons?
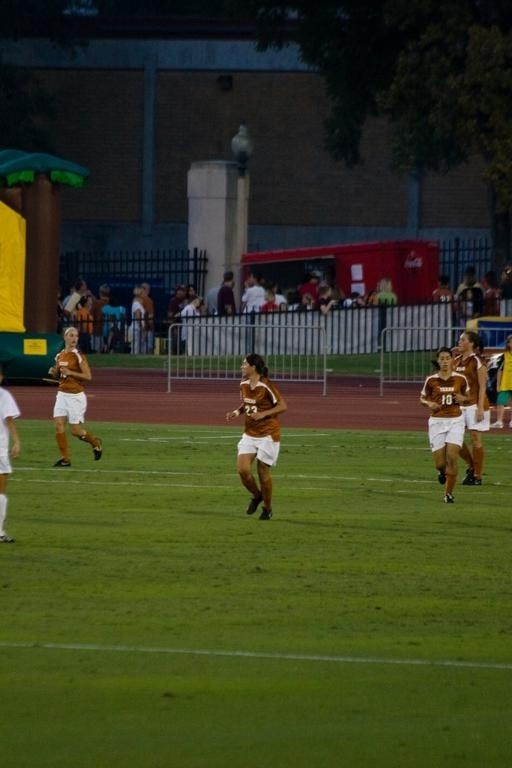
[170,268,397,355]
[47,325,102,467]
[418,345,471,503]
[451,330,490,485]
[1,369,22,543]
[224,353,287,522]
[432,263,512,334]
[59,278,154,354]
[490,334,511,429]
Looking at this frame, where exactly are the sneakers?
[53,459,70,466]
[439,472,446,484]
[463,468,481,484]
[246,495,263,513]
[0,535,13,542]
[260,506,272,519]
[444,495,454,502]
[93,437,102,460]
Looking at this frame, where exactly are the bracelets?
[232,409,241,417]
